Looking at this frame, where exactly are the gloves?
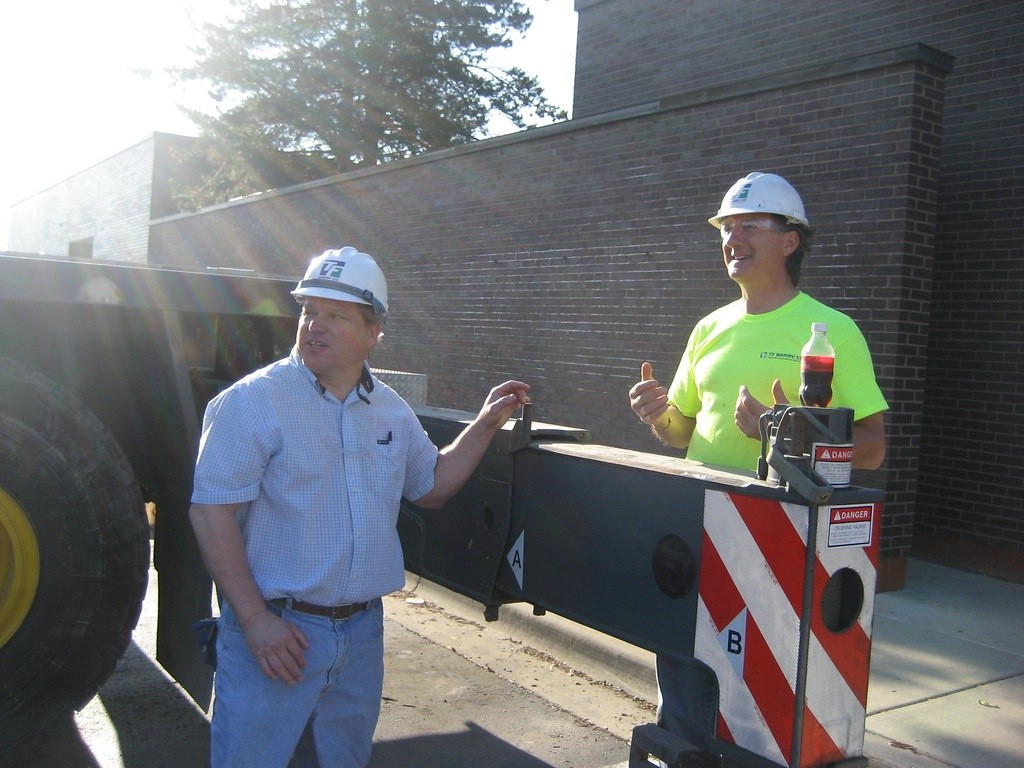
[193,617,219,672]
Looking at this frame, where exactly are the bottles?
[798,323,836,407]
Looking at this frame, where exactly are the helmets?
[707,172,808,231]
[290,246,389,317]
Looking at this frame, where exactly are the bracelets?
[651,404,672,431]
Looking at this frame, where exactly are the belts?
[270,596,378,621]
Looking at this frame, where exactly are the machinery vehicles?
[0,252,885,767]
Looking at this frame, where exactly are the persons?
[630,172,890,768]
[189,246,531,768]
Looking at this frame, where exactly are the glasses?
[720,216,794,239]
